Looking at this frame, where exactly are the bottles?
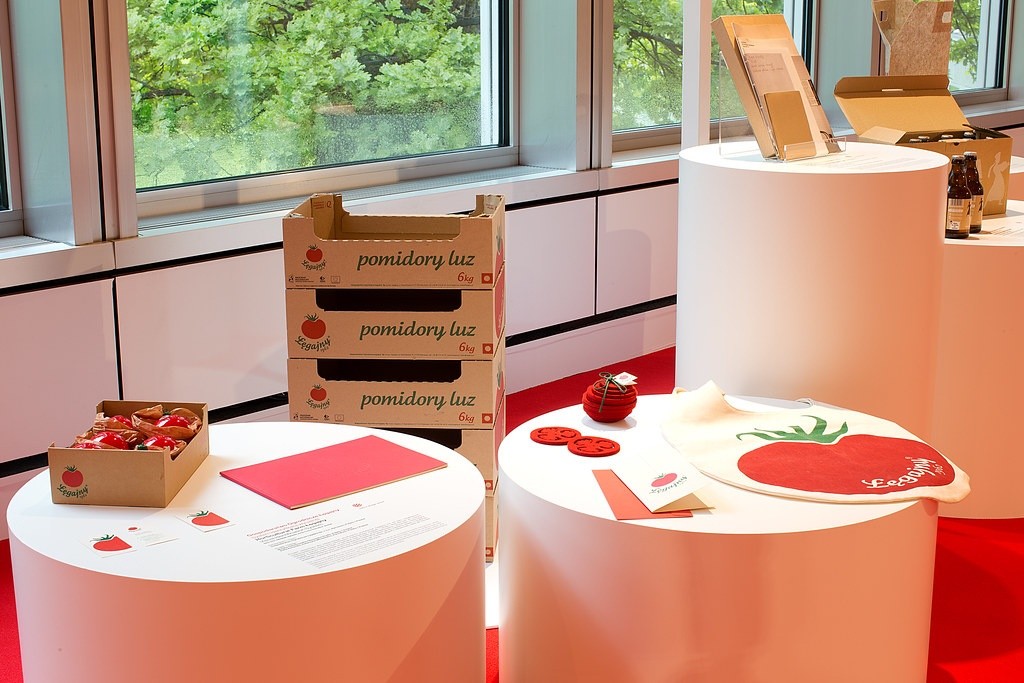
[945,155,971,239]
[910,132,994,142]
[964,152,983,233]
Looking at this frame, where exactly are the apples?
[70,414,189,455]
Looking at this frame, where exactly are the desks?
[498,391,940,683]
[675,137,950,450]
[6,421,486,683]
[936,196,1024,520]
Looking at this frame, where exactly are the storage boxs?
[456,389,506,497]
[48,399,210,508]
[831,75,1012,216]
[286,260,506,360]
[711,13,843,158]
[286,324,505,429]
[281,193,507,291]
[485,477,499,563]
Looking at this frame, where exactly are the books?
[709,12,841,159]
